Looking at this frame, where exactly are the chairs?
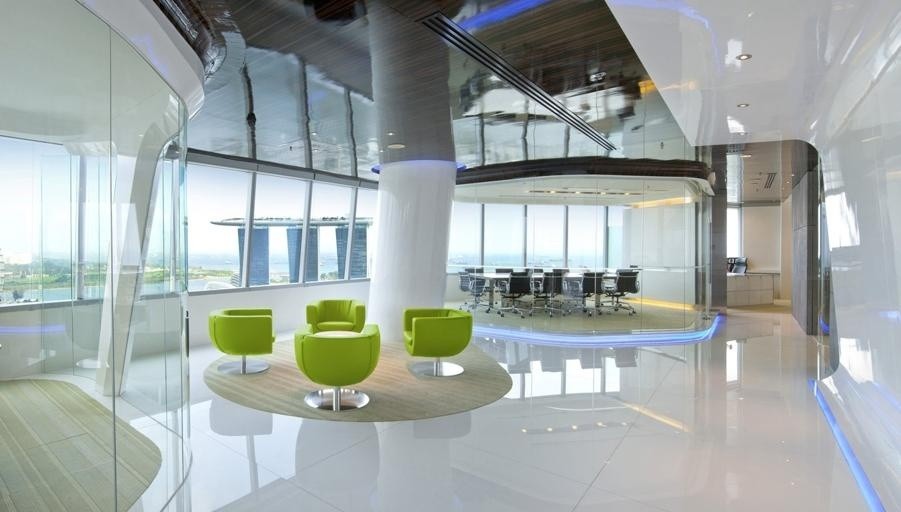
[459,266,641,318]
[208,299,473,412]
[732,258,748,272]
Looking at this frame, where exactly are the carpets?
[0,378,163,511]
[205,337,513,422]
